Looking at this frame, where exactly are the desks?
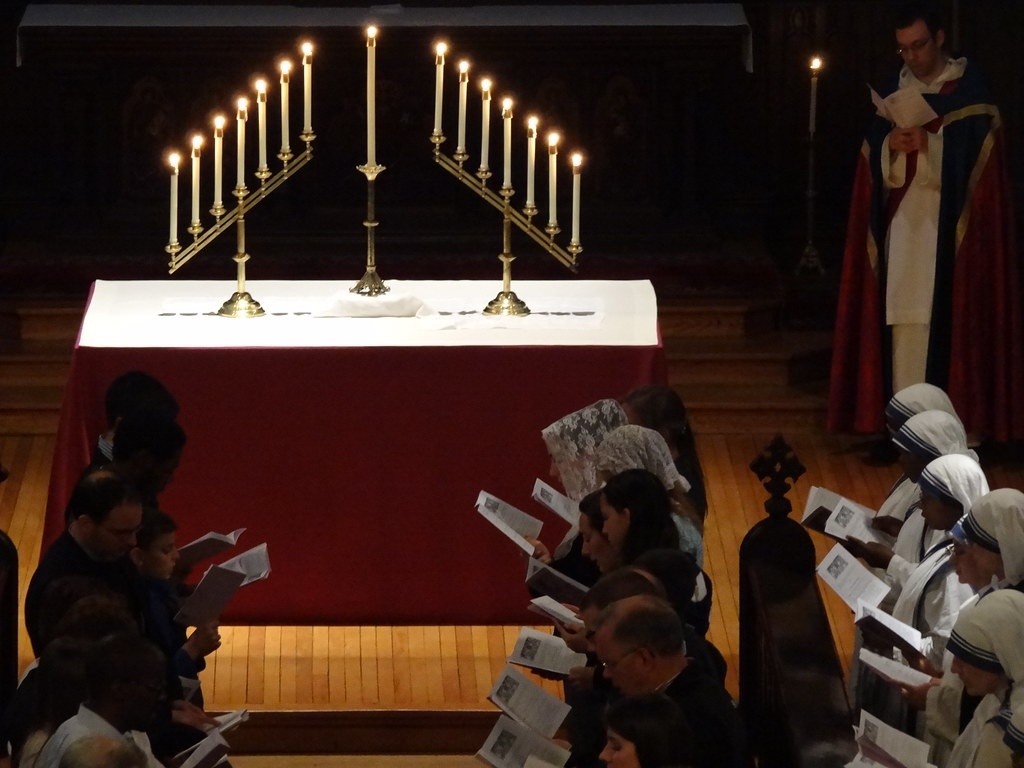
[15,4,771,258]
[38,279,709,627]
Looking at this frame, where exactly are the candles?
[365,26,379,168]
[480,79,491,170]
[501,97,513,190]
[236,97,248,188]
[168,154,178,245]
[280,60,292,154]
[809,58,821,133]
[191,136,201,224]
[571,154,581,246]
[303,43,313,134]
[433,43,445,134]
[457,61,468,154]
[257,79,267,172]
[548,133,558,225]
[213,117,224,207]
[526,117,537,206]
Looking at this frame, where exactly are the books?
[866,80,939,132]
[473,478,588,768]
[168,528,271,768]
[799,485,935,768]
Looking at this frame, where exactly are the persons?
[827,8,1024,467]
[0,370,220,768]
[524,383,755,768]
[846,383,1024,768]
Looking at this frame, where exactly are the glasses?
[100,522,144,538]
[950,539,968,560]
[918,491,939,509]
[895,34,932,58]
[602,648,638,674]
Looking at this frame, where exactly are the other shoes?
[864,441,902,466]
